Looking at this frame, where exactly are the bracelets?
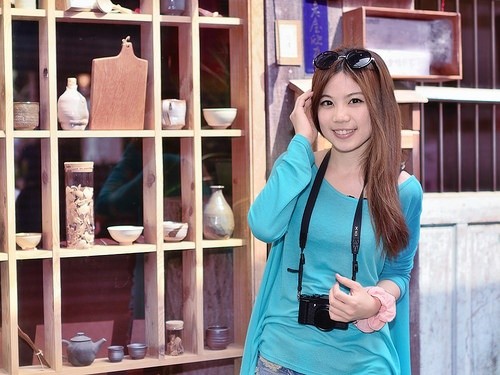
[353,286,397,334]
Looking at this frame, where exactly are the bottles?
[57,77,89,131]
[203,186,234,239]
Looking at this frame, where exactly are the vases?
[203,186,234,240]
[59,77,90,131]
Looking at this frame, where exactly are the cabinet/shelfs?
[288,7,463,191]
[0,0,267,374]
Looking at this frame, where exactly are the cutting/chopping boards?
[88,41,148,130]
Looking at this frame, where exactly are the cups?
[161,99,186,130]
[127,343,148,360]
[107,346,124,362]
[13,102,40,130]
[160,0,186,15]
[206,324,230,350]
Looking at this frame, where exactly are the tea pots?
[62,332,106,367]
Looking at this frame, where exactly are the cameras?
[298,293,348,332]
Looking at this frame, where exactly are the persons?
[93,45,234,351]
[238,47,424,375]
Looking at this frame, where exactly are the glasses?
[313,49,376,70]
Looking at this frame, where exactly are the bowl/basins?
[16,233,42,250]
[164,221,189,242]
[202,108,238,129]
[106,225,144,245]
